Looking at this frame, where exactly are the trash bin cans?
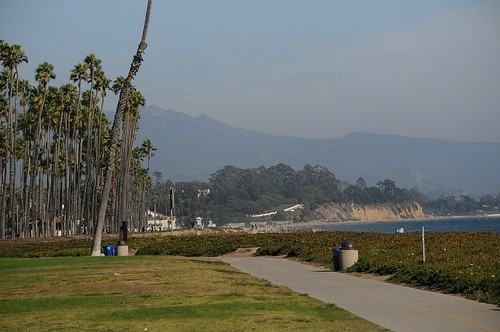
[332,247,342,270]
[102,244,116,256]
